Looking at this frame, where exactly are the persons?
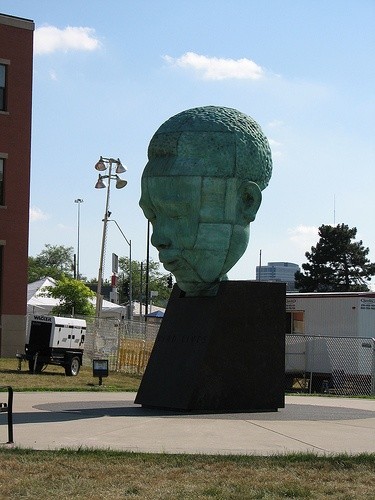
[140,105,271,294]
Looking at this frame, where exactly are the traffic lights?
[167,276,173,288]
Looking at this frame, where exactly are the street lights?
[101,219,134,337]
[75,199,83,278]
[94,156,128,362]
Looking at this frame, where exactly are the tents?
[123,302,166,321]
[27,277,126,359]
[144,311,164,324]
